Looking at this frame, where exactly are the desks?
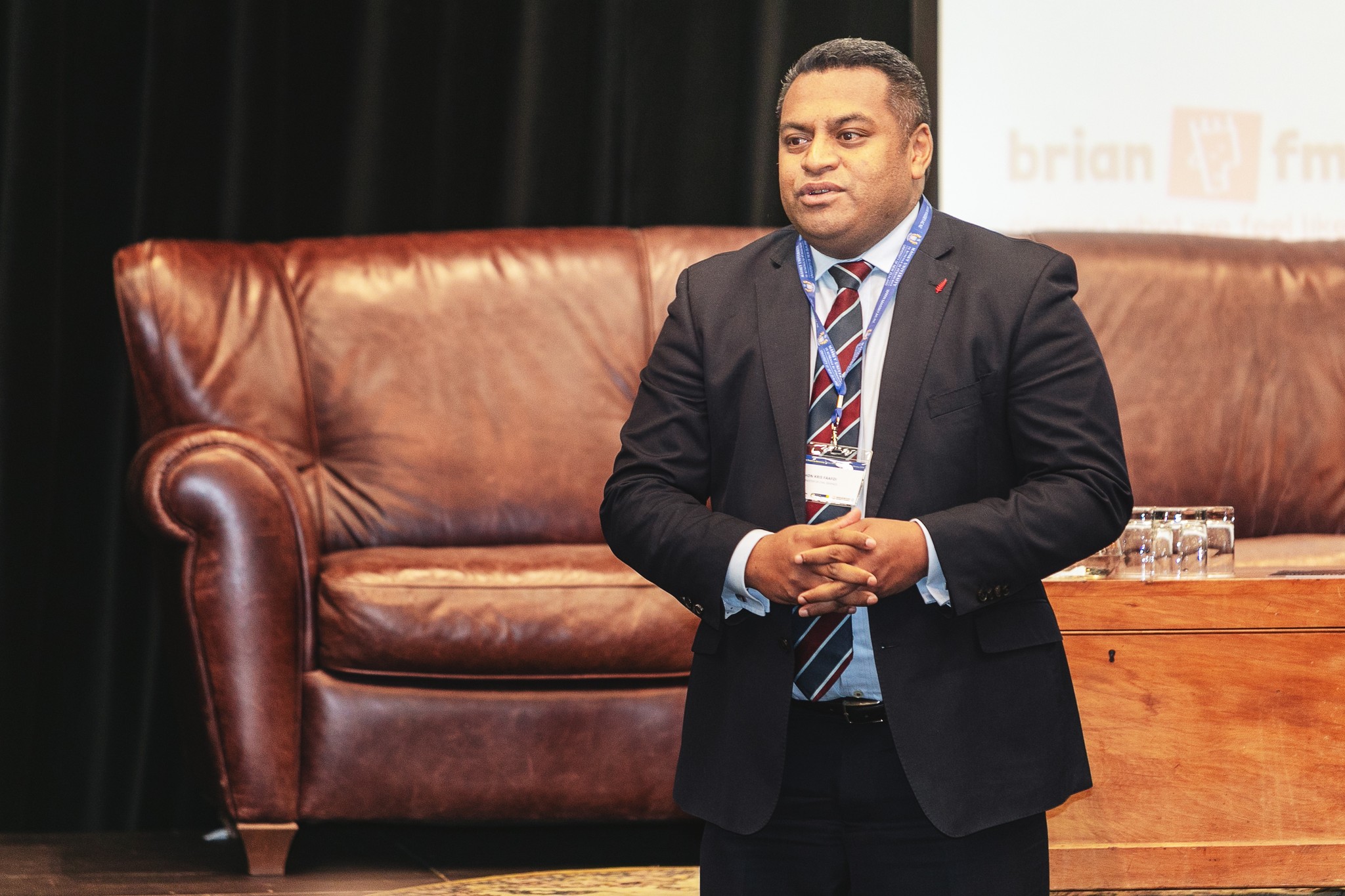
[1039,574,1345,891]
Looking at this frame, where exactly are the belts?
[797,699,887,723]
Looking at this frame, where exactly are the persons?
[598,36,1138,896]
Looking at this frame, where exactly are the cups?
[1118,507,1182,581]
[1181,506,1235,578]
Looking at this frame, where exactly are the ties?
[794,256,874,701]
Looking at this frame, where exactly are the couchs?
[111,223,1345,880]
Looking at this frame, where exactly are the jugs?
[1050,539,1122,579]
